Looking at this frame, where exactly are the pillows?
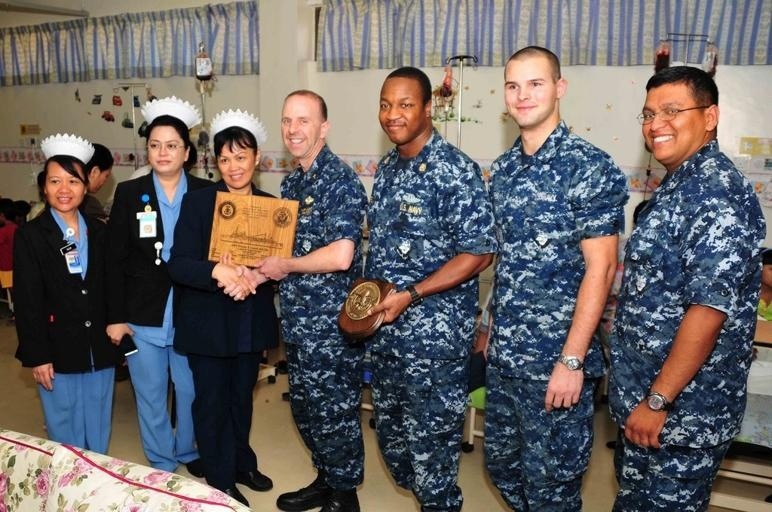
[0,426,58,511]
[48,446,250,512]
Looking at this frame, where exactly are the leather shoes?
[186,458,362,512]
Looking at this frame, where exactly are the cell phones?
[119,333,139,357]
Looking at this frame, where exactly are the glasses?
[638,106,711,127]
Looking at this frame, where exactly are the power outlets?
[733,156,750,171]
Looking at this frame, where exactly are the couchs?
[709,343,772,512]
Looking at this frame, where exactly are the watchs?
[557,355,583,370]
[646,392,674,412]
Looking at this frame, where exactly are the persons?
[105,96,215,478]
[0,198,32,320]
[490,45,627,512]
[754,246,772,318]
[607,65,767,512]
[363,67,496,512]
[217,90,366,511]
[12,133,115,455]
[166,108,279,508]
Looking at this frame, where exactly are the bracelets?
[407,286,423,307]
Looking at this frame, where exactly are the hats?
[140,96,203,129]
[39,133,95,165]
[209,108,268,158]
